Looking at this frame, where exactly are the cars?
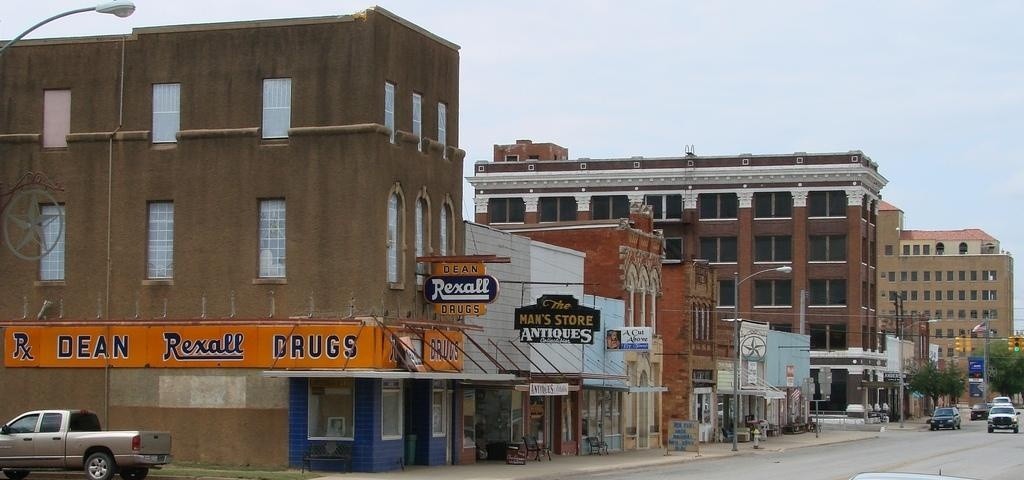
[969,396,1021,433]
[931,408,961,430]
[849,472,971,479]
[477,409,522,459]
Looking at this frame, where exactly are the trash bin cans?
[405,434,417,465]
[745,415,755,441]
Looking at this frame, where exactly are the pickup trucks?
[1,408,172,479]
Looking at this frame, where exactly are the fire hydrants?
[751,428,762,450]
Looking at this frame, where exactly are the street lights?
[732,266,792,452]
[0,0,135,54]
[899,319,942,428]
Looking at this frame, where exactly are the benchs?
[587,437,609,455]
[521,436,552,461]
[301,443,353,474]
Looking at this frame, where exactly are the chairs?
[721,428,733,443]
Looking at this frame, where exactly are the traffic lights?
[954,337,972,352]
[1008,337,1024,353]
